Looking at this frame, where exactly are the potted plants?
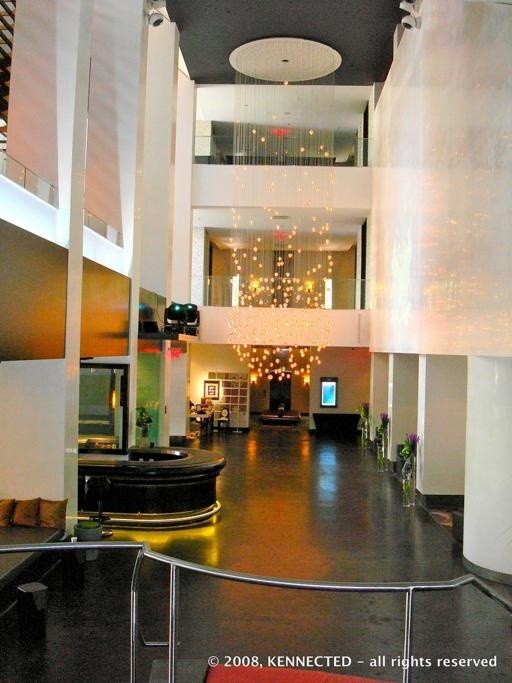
[75,520,104,559]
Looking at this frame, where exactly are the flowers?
[359,400,420,508]
[136,409,152,438]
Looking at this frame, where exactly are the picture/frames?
[204,381,219,399]
[319,376,338,408]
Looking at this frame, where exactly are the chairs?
[217,408,229,433]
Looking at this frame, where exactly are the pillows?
[0,499,67,532]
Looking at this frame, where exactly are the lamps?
[225,33,342,385]
[160,303,202,340]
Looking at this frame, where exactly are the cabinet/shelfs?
[213,379,250,435]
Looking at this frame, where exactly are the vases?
[139,423,149,448]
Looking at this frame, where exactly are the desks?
[191,411,213,438]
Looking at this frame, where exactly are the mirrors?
[79,362,130,456]
